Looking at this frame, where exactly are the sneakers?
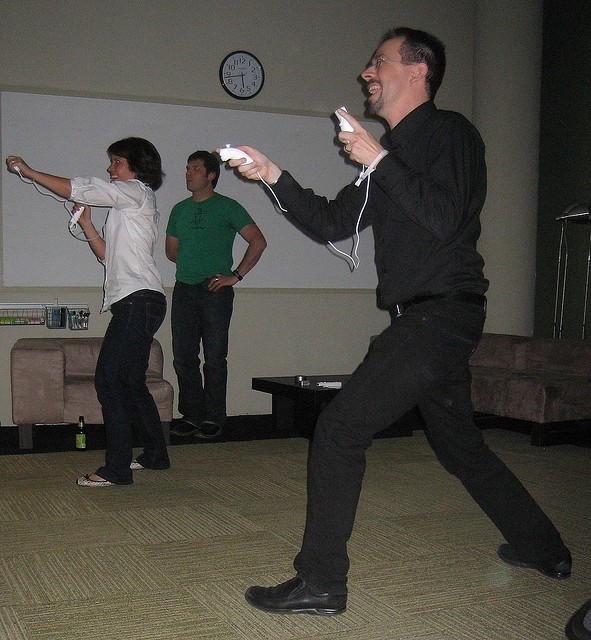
[168,417,195,437]
[128,457,144,470]
[193,417,222,438]
[75,472,117,487]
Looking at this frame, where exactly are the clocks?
[218,50,265,101]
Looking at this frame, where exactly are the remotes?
[315,380,344,392]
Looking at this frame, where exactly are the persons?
[166,150,267,438]
[225,26,572,615]
[6,138,171,485]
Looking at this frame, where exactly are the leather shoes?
[495,540,572,579]
[243,578,347,617]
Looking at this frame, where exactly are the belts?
[388,289,485,317]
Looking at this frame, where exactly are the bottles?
[75,415,87,452]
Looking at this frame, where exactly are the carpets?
[0,427,590,640]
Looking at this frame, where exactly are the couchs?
[9,337,174,450]
[469,332,590,445]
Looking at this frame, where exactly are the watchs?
[232,269,243,283]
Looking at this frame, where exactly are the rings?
[11,159,16,165]
[344,144,352,153]
[214,277,219,283]
